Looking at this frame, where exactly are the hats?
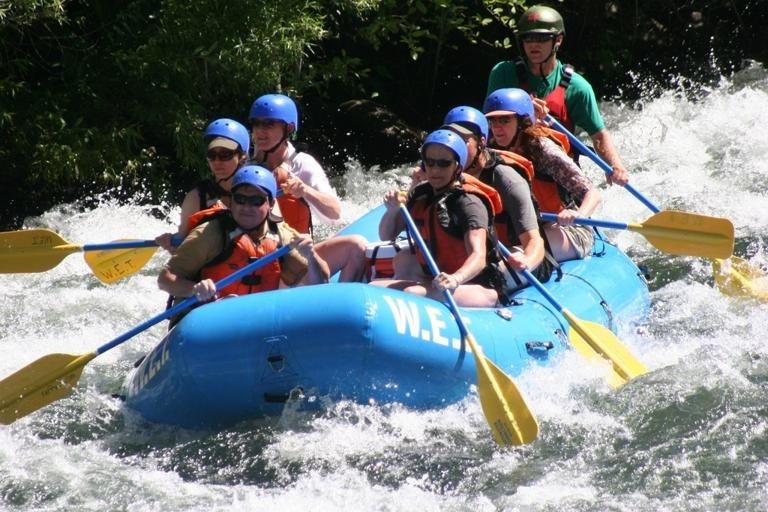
[207,136,240,152]
[441,123,480,136]
[485,110,518,116]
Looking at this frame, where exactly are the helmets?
[444,105,489,141]
[249,94,298,131]
[483,88,534,128]
[518,6,565,35]
[204,118,251,153]
[422,130,468,170]
[230,166,277,200]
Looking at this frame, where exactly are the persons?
[481,89,601,263]
[482,2,628,187]
[440,104,546,294]
[156,118,287,255]
[245,94,369,283]
[156,165,330,326]
[369,128,507,309]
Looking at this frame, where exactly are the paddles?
[489,235,644,390]
[84,178,291,284]
[0,239,296,425]
[545,115,768,306]
[2,228,184,273]
[396,191,539,447]
[541,210,733,259]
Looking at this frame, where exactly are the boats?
[125,202,649,430]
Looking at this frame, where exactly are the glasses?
[490,117,512,125]
[523,34,554,43]
[252,119,281,128]
[234,192,266,207]
[426,158,454,168]
[206,151,237,161]
